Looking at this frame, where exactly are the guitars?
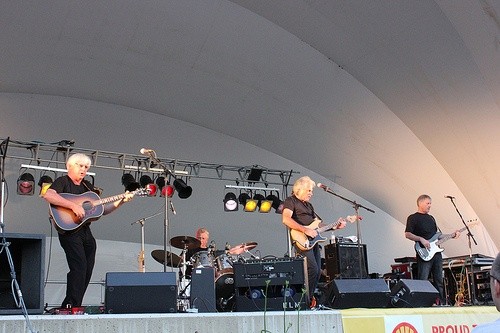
[414,218,479,263]
[290,215,360,252]
[48,187,150,236]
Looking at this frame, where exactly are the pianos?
[441,254,496,306]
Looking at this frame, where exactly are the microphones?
[444,194,455,198]
[317,183,332,190]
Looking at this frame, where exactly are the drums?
[190,250,217,277]
[214,254,238,283]
[215,272,236,312]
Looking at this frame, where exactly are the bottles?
[330,231,336,244]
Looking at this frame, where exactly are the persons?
[179,227,218,282]
[43,153,134,307]
[490,251,500,312]
[405,194,460,304]
[282,176,346,309]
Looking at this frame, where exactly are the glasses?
[486,272,500,285]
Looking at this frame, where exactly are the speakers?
[104,272,177,314]
[323,279,391,310]
[325,244,368,279]
[191,268,216,313]
[386,279,439,308]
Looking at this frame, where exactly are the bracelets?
[303,227,306,233]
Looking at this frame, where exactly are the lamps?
[121,170,192,199]
[17,167,36,196]
[37,170,57,198]
[253,189,273,213]
[238,188,258,213]
[223,187,238,212]
[266,190,284,215]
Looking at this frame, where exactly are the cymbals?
[169,235,202,249]
[151,250,184,268]
[227,241,258,255]
